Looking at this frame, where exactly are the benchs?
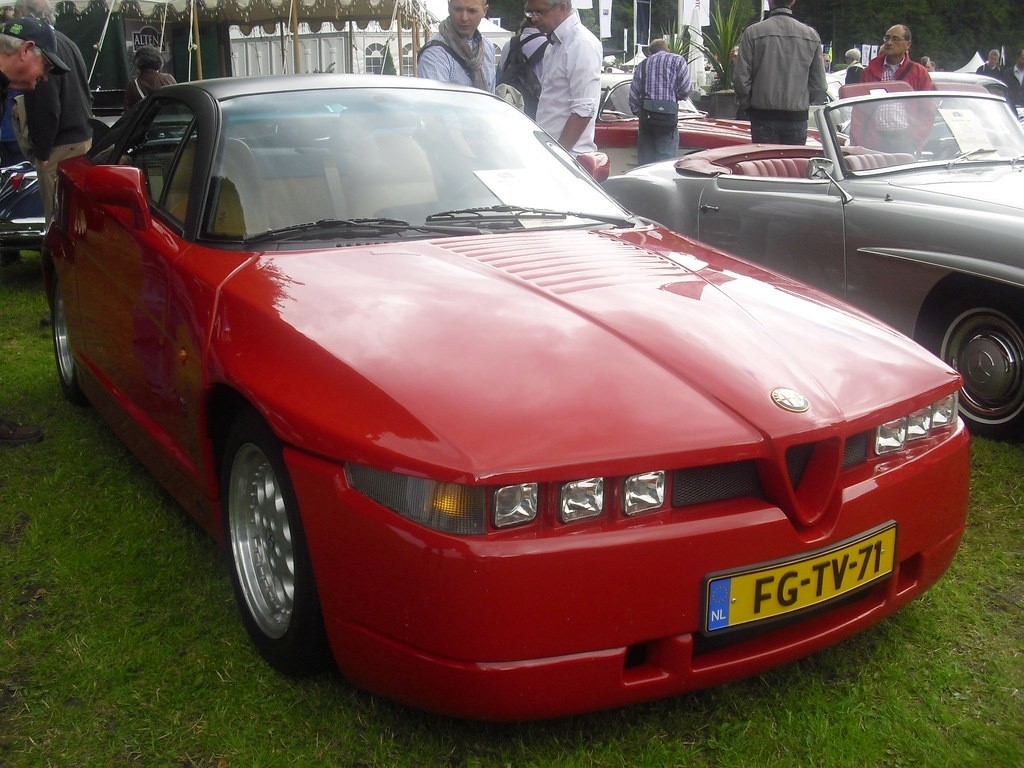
[733,150,916,182]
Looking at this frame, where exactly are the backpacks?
[495,32,554,123]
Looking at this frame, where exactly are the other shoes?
[40,317,53,329]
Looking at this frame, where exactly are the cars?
[593,72,851,178]
[35,70,974,715]
[592,73,700,121]
[0,102,350,251]
[841,71,1024,152]
[594,90,1024,434]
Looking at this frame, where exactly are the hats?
[0,16,71,76]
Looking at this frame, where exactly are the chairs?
[337,129,490,225]
[170,135,286,238]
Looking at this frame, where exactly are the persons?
[628,38,692,166]
[14,0,94,224]
[122,45,177,117]
[825,48,1024,110]
[731,1,828,145]
[417,0,496,97]
[851,24,937,157]
[494,3,553,121]
[0,16,71,167]
[524,0,603,163]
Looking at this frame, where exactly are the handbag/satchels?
[642,98,679,133]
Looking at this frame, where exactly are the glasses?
[884,37,906,42]
[524,4,557,20]
[37,45,55,74]
